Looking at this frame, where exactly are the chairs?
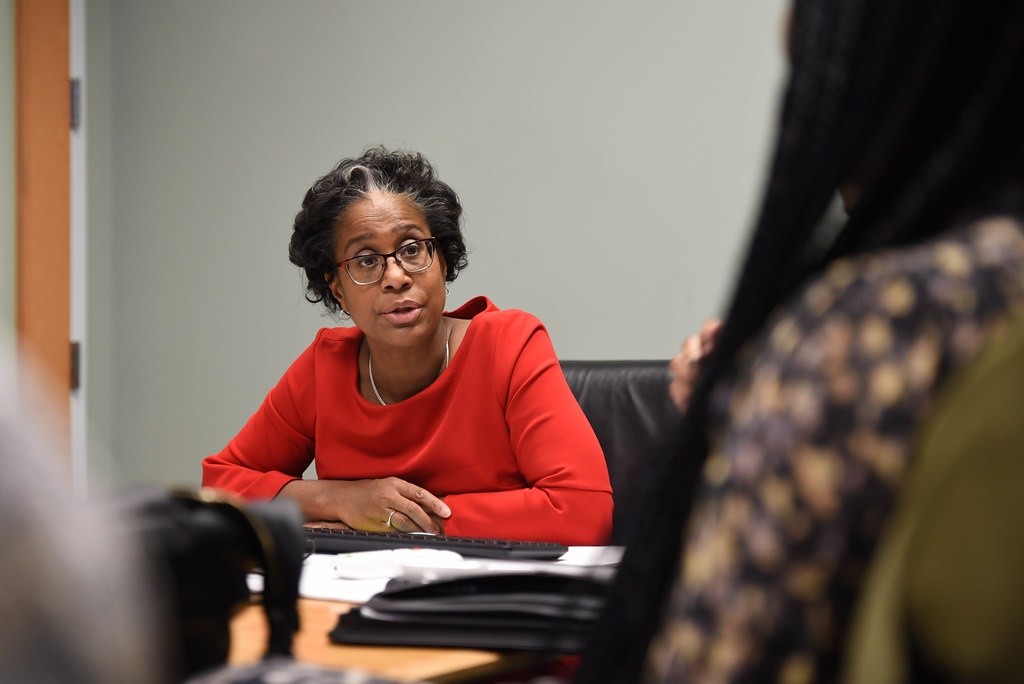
[555,360,676,546]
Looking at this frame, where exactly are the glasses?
[330,236,436,285]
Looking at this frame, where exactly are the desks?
[228,584,618,684]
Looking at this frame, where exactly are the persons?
[198,147,618,549]
[577,48,1024,684]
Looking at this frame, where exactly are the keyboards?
[307,525,569,563]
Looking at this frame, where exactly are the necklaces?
[367,326,451,408]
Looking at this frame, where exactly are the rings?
[386,510,396,530]
